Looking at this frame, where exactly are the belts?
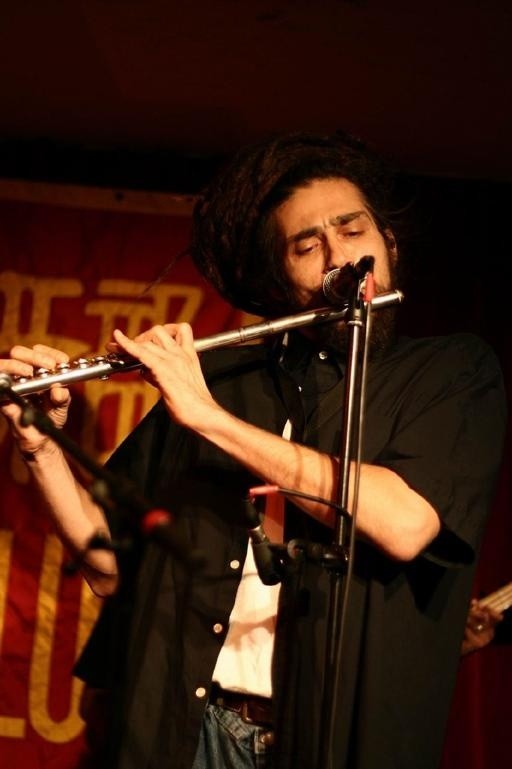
[208,691,274,726]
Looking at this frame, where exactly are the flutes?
[10,288,404,396]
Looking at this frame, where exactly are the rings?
[475,622,487,635]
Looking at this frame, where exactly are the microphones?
[320,255,377,304]
[246,500,288,586]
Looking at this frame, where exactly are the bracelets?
[18,438,51,458]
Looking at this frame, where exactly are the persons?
[1,135,504,768]
[456,600,504,657]
